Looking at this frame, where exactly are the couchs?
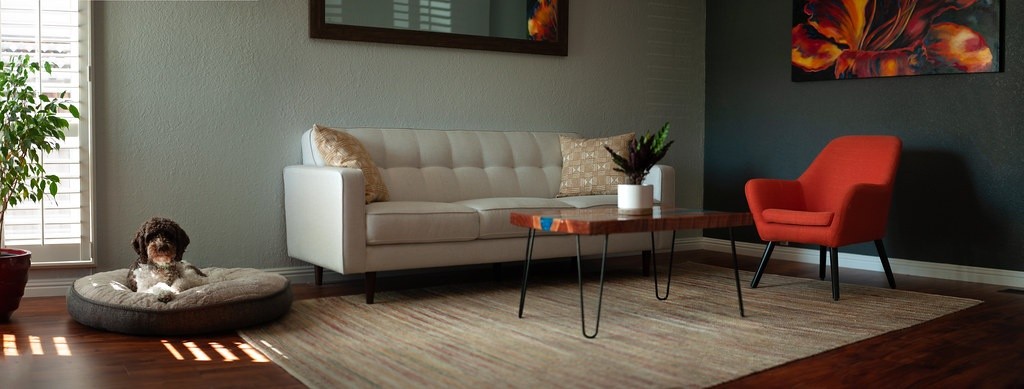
[281,130,675,306]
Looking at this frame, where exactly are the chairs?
[744,135,900,300]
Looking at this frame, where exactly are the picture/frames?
[309,0,571,57]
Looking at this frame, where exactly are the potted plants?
[611,123,676,213]
[0,54,83,323]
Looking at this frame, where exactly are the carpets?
[235,256,985,389]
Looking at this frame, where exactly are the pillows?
[312,122,391,203]
[555,134,636,196]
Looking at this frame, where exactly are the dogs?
[127,216,208,302]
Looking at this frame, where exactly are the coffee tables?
[511,209,753,339]
